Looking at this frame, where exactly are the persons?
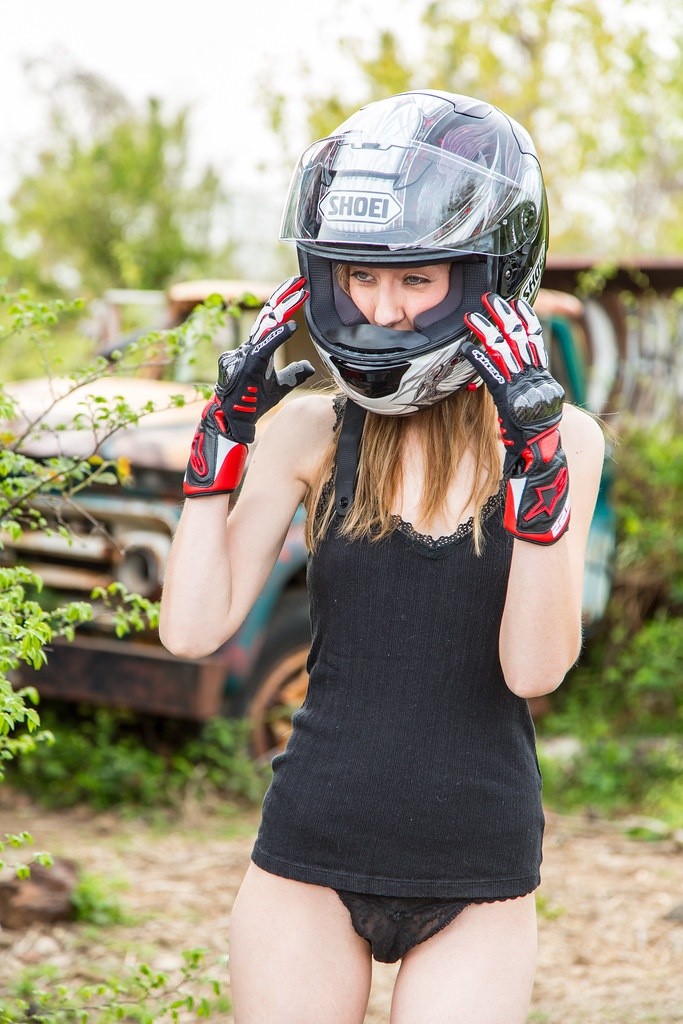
[158,90,605,1024]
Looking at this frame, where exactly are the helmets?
[279,89,549,417]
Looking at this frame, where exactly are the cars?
[0,281,617,782]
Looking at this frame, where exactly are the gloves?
[462,292,571,545]
[182,275,316,499]
